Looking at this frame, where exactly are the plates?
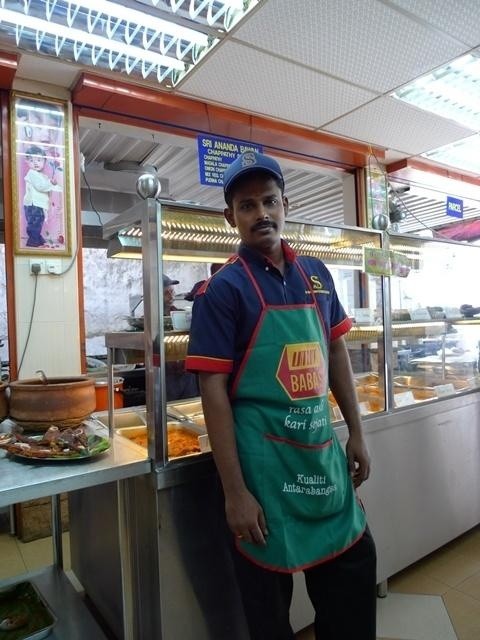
[8,434,111,461]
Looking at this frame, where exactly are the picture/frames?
[10,89,73,257]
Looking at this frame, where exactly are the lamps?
[106,234,364,272]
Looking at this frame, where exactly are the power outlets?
[29,258,62,275]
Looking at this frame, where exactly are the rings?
[237,535,244,540]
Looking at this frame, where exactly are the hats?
[223,150,284,193]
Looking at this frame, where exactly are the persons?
[15,100,63,157]
[181,148,379,640]
[118,271,200,406]
[20,145,64,247]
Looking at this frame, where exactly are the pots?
[0,375,97,433]
